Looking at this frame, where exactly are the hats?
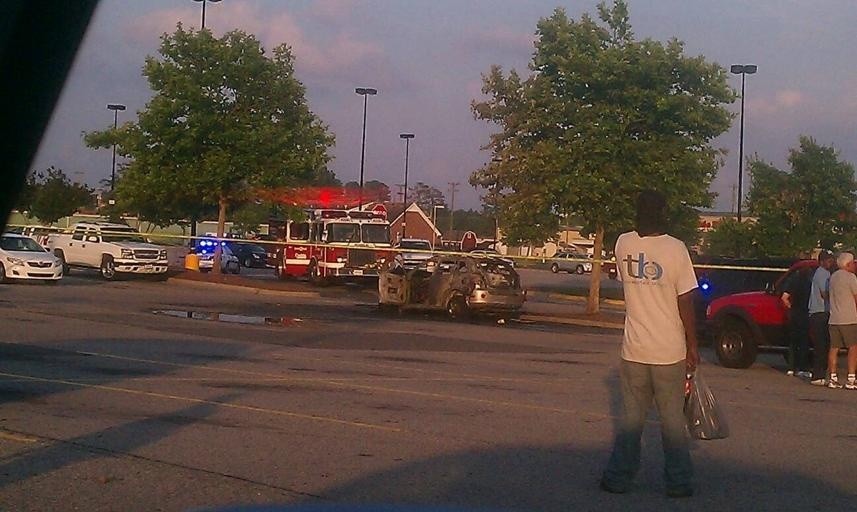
[634,191,664,234]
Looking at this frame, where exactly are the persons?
[600,188,700,499]
[781,264,817,378]
[828,253,857,390]
[807,250,833,385]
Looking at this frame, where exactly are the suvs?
[395,237,437,276]
[704,259,857,370]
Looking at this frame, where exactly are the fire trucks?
[267,201,398,287]
[228,224,276,267]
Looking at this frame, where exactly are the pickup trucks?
[42,221,169,280]
[14,224,60,250]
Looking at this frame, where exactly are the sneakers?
[845,380,857,389]
[810,378,825,385]
[828,378,843,389]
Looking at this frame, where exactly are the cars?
[471,250,514,270]
[186,240,240,274]
[376,253,525,319]
[220,242,267,267]
[0,231,64,284]
[548,250,617,280]
[691,257,812,348]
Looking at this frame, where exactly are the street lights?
[353,85,378,217]
[398,132,412,238]
[446,181,461,236]
[106,104,127,189]
[433,206,445,249]
[731,64,757,254]
[193,1,222,247]
